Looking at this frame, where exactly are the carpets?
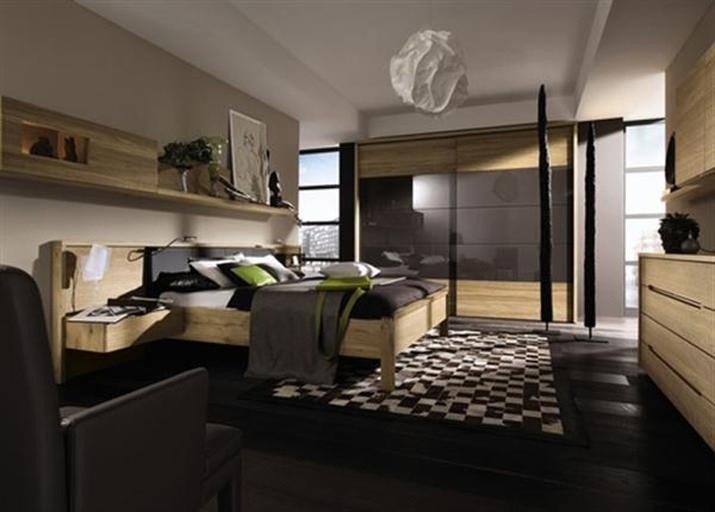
[259,326,591,449]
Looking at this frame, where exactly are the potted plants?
[656,209,701,254]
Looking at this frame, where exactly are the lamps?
[390,1,471,115]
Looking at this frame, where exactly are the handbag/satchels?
[315,276,373,292]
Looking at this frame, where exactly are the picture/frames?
[229,108,275,205]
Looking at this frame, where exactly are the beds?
[142,247,452,394]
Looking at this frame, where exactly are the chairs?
[1,266,247,512]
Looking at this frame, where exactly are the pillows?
[190,252,302,289]
[316,257,381,293]
[419,253,450,267]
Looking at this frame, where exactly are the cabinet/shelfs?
[637,254,715,454]
[676,1,715,193]
